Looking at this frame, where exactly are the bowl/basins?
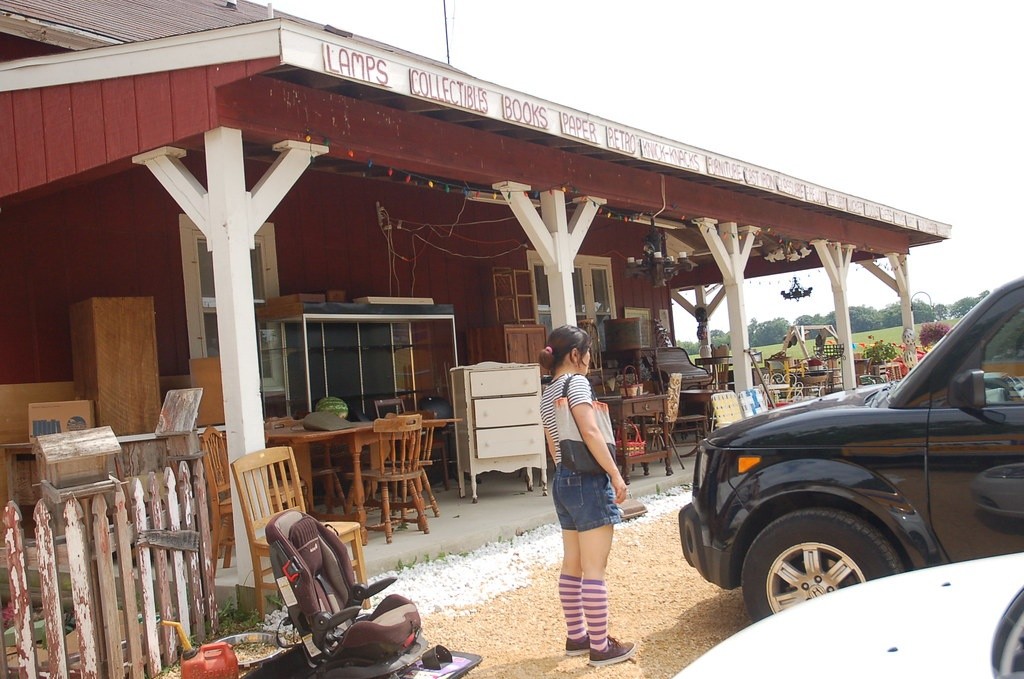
[214,632,287,666]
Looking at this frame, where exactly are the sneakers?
[587,634,637,666]
[564,635,591,656]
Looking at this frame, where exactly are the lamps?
[625,218,693,287]
[781,277,812,300]
[765,247,811,262]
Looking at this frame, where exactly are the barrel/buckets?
[161,620,239,679]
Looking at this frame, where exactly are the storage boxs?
[28,401,93,438]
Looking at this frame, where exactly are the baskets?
[619,365,644,399]
[614,417,647,458]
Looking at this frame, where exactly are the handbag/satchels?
[555,374,618,475]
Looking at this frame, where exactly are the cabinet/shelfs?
[450,322,551,505]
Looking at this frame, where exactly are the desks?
[265,418,462,546]
[598,395,670,483]
[681,388,730,457]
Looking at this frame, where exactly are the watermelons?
[315,396,348,420]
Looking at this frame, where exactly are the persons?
[538,325,636,667]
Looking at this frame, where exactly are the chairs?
[764,356,873,387]
[202,396,455,622]
[650,346,714,467]
[578,319,606,395]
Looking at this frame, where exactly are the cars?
[678,277,1023,622]
[669,552,1024,679]
